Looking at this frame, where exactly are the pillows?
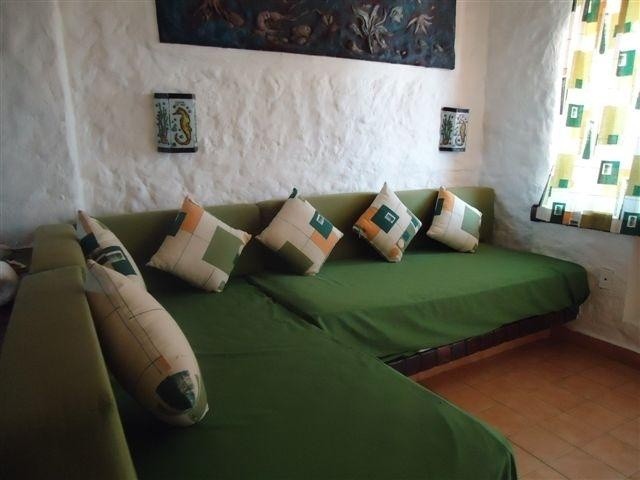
[426,184,484,254]
[83,258,210,430]
[146,195,252,293]
[74,207,148,293]
[256,186,343,276]
[350,181,424,265]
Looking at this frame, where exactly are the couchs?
[1,183,591,479]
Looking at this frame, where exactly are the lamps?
[439,105,471,152]
[153,91,199,153]
